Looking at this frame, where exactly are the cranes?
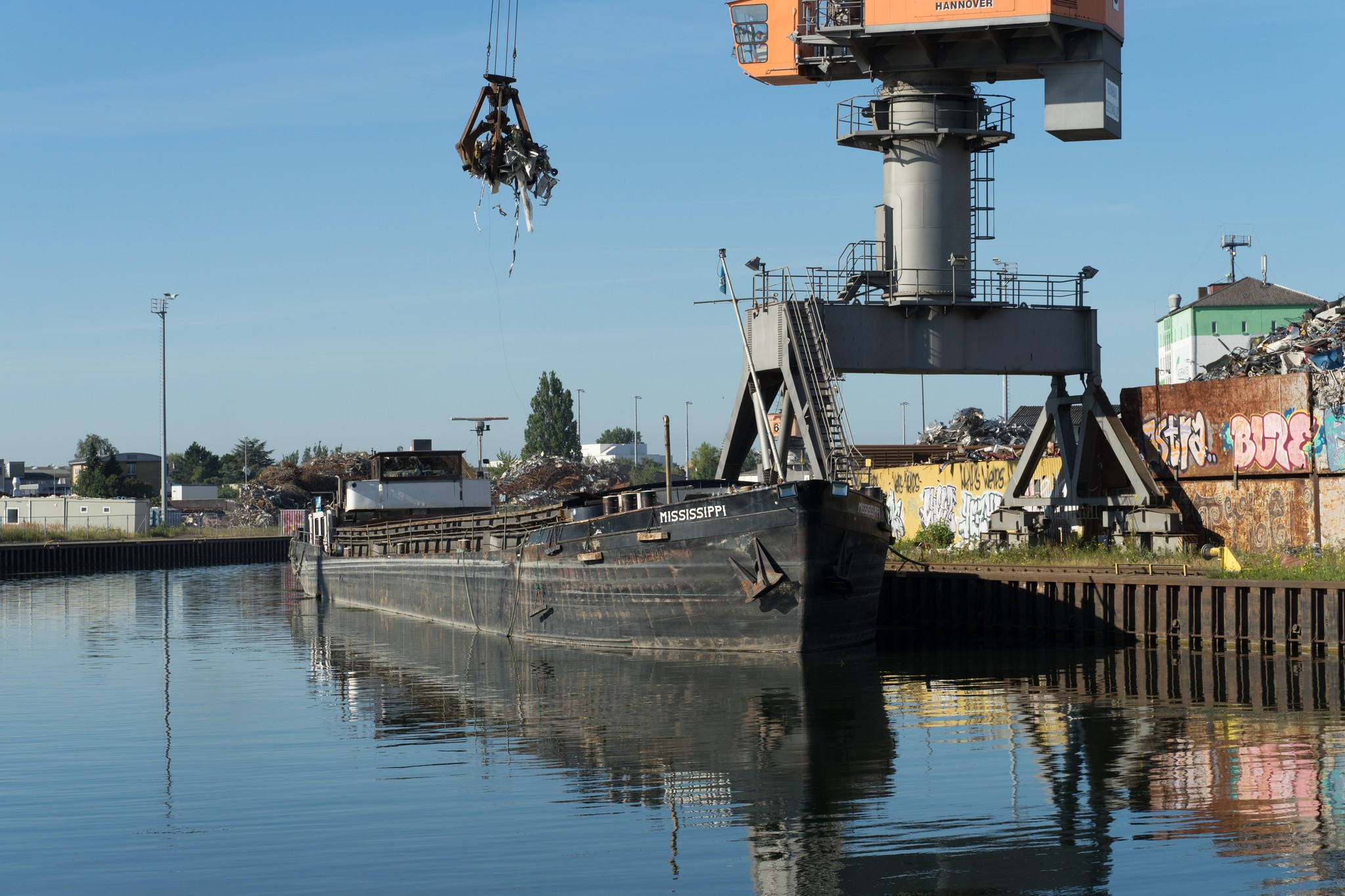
[457,0,1199,532]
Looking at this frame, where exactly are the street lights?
[682,402,693,480]
[152,293,181,526]
[574,389,586,461]
[993,258,1019,422]
[631,396,642,468]
[53,464,56,495]
[898,403,909,445]
[243,436,248,484]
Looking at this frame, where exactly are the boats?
[289,249,893,655]
